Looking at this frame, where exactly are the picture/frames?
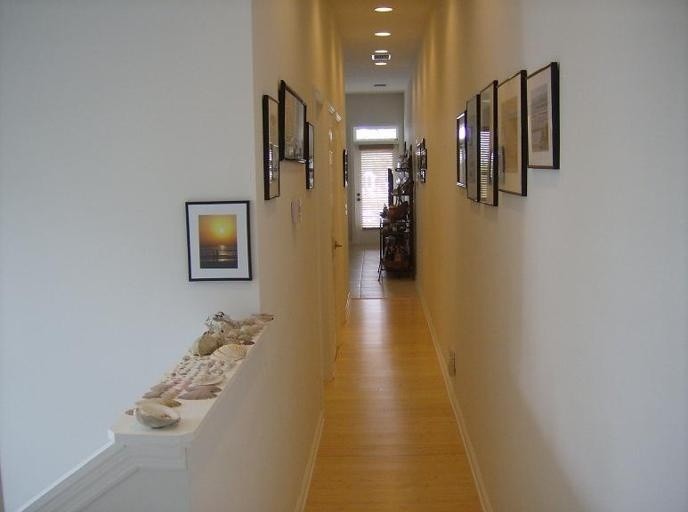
[262,80,316,200]
[183,200,251,282]
[456,62,558,207]
[415,138,427,182]
[343,149,349,187]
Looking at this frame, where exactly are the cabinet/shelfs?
[378,166,414,279]
